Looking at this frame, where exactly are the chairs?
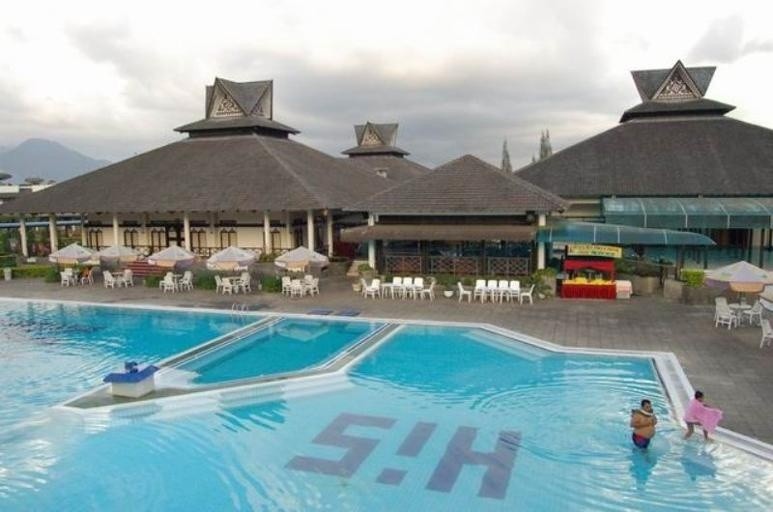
[279,273,323,300]
[355,273,437,301]
[60,265,95,288]
[101,267,135,290]
[214,271,254,297]
[712,295,773,349]
[451,277,539,306]
[157,268,195,294]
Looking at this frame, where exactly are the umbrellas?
[146,245,195,261]
[48,241,95,258]
[704,260,773,284]
[274,245,329,265]
[95,243,141,257]
[207,245,256,261]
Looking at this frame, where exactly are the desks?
[561,280,616,299]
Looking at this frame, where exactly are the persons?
[681,390,708,439]
[630,399,657,449]
